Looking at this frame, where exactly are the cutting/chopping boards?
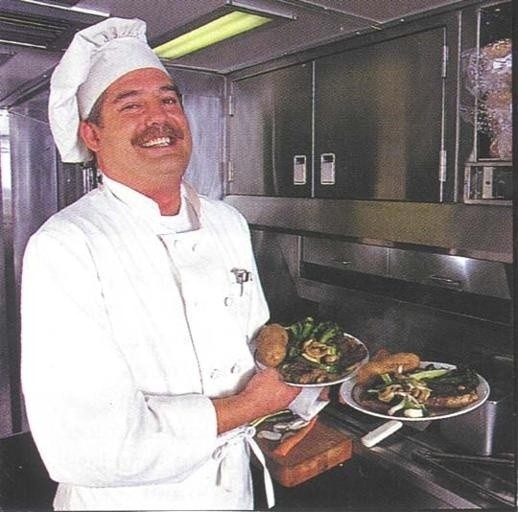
[250,418,353,488]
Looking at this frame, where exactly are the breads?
[423,392,479,407]
[256,323,289,366]
[357,350,419,385]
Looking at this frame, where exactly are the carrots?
[274,415,319,455]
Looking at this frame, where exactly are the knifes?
[361,422,432,449]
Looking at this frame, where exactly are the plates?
[254,332,369,388]
[340,361,491,422]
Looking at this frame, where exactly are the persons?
[20,17,389,511]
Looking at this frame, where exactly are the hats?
[48,16,170,164]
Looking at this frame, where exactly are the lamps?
[152,10,273,60]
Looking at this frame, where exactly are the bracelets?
[328,383,341,407]
